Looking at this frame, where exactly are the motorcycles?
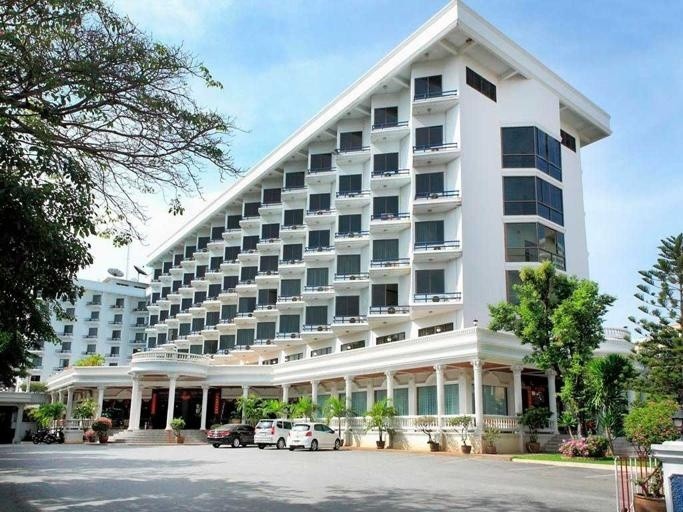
[32,426,65,444]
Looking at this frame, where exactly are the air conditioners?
[57,146,444,371]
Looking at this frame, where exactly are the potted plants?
[480,423,502,454]
[620,397,683,512]
[91,415,112,444]
[516,405,555,454]
[414,413,447,453]
[73,396,99,442]
[363,396,398,449]
[168,415,186,444]
[322,394,358,446]
[84,429,96,442]
[450,412,475,455]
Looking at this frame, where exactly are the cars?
[285,421,341,450]
[207,423,254,448]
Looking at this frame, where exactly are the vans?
[254,418,294,449]
